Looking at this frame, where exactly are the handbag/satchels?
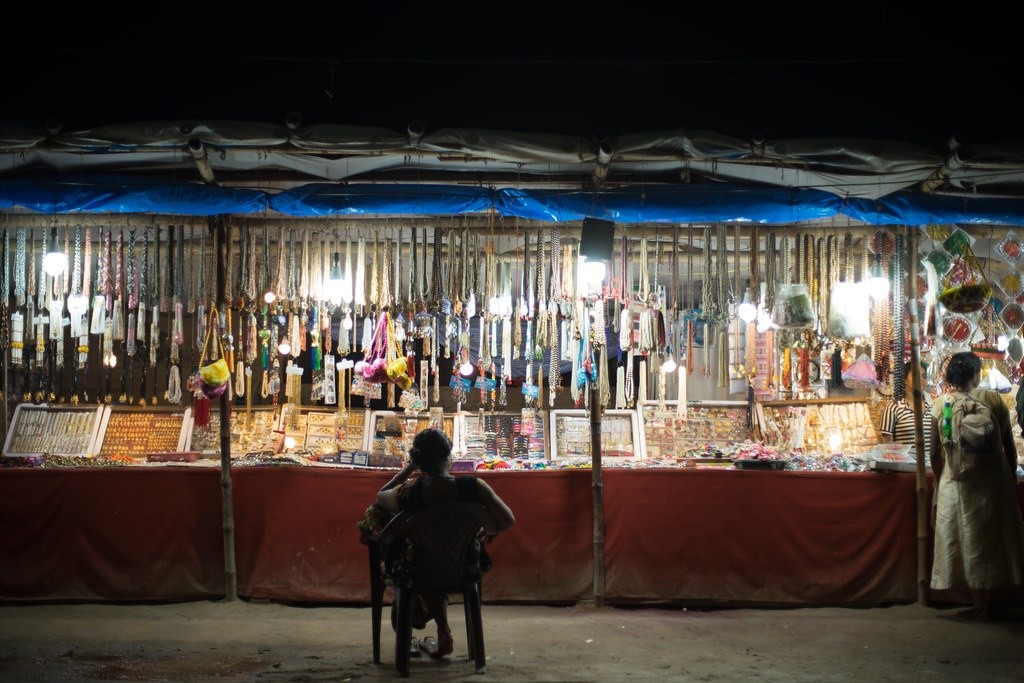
[978,362,1013,394]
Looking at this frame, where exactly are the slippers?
[409,635,442,659]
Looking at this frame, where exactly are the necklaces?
[817,403,874,456]
[0,220,906,410]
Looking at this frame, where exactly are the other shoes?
[962,605,1004,623]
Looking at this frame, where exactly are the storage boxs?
[1,390,917,472]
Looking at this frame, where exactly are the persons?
[364,428,517,659]
[879,361,938,460]
[929,353,1024,620]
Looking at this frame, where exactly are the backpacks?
[938,387,1004,482]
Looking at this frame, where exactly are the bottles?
[942,403,953,439]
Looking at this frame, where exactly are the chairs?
[360,475,516,674]
